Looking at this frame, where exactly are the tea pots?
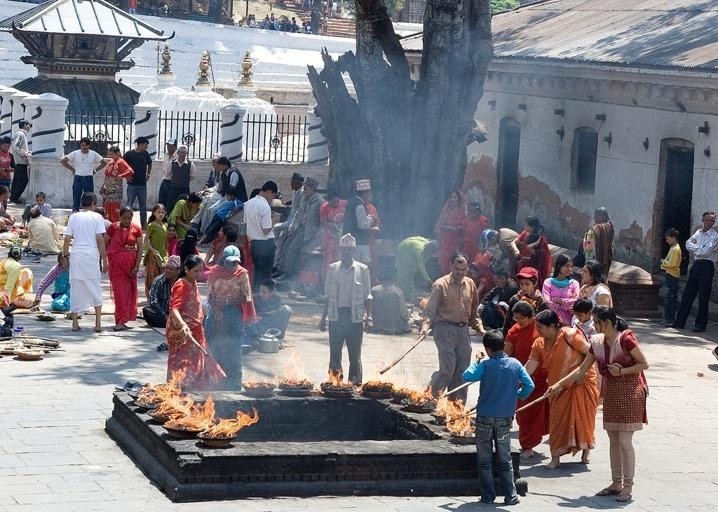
[259,328,281,352]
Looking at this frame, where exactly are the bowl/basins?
[116,384,237,447]
[391,390,476,444]
[243,381,394,398]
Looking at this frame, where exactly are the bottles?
[14,326,24,336]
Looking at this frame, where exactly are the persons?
[659,211,718,332]
[395,189,649,507]
[268,173,383,385]
[126,1,338,35]
[2,116,291,394]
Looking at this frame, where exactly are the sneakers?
[504,493,522,506]
[544,460,562,471]
[271,270,283,277]
[691,327,706,333]
[276,272,294,281]
[581,455,590,464]
[520,449,535,459]
[658,319,685,330]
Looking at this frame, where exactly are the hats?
[177,145,189,153]
[222,244,242,264]
[304,176,320,187]
[515,265,541,281]
[477,228,494,252]
[338,232,358,249]
[166,254,182,270]
[165,137,178,146]
[292,172,305,181]
[355,178,372,191]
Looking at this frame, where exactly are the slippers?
[113,324,129,331]
[93,326,103,332]
[596,487,623,497]
[614,491,633,502]
[72,325,82,331]
